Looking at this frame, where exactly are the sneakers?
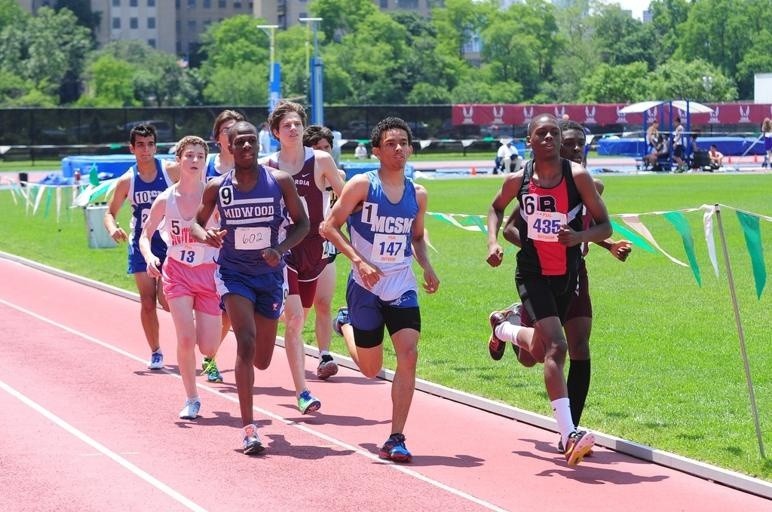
[201,356,223,383]
[489,311,506,361]
[332,307,349,336]
[149,348,164,370]
[179,399,200,419]
[507,304,522,325]
[559,428,593,457]
[297,391,321,415]
[243,432,264,455]
[316,355,338,380]
[564,431,595,466]
[379,434,412,462]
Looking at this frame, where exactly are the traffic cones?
[728,156,732,164]
[754,154,759,163]
[470,166,478,175]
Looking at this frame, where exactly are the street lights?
[297,15,324,128]
[255,23,280,156]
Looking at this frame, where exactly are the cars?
[34,117,176,141]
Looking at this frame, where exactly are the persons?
[104,109,348,378]
[504,118,635,457]
[355,143,367,155]
[258,100,346,415]
[487,113,612,466]
[139,135,222,418]
[321,117,439,462]
[760,118,772,167]
[642,118,724,172]
[189,120,311,454]
